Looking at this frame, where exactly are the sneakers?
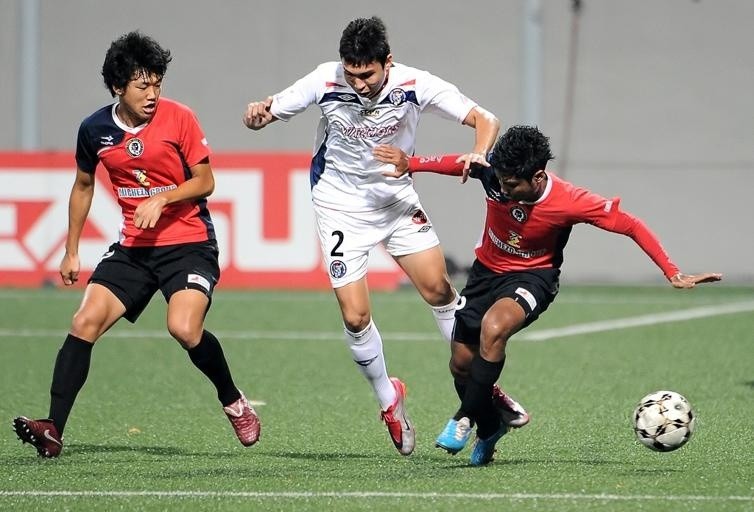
[11,415,62,458]
[435,418,472,455]
[221,387,261,446]
[378,377,415,455]
[491,384,529,428]
[469,416,507,466]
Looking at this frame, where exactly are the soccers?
[633,391,695,452]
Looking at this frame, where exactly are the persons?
[371,124,723,466]
[243,18,501,456]
[11,33,261,460]
